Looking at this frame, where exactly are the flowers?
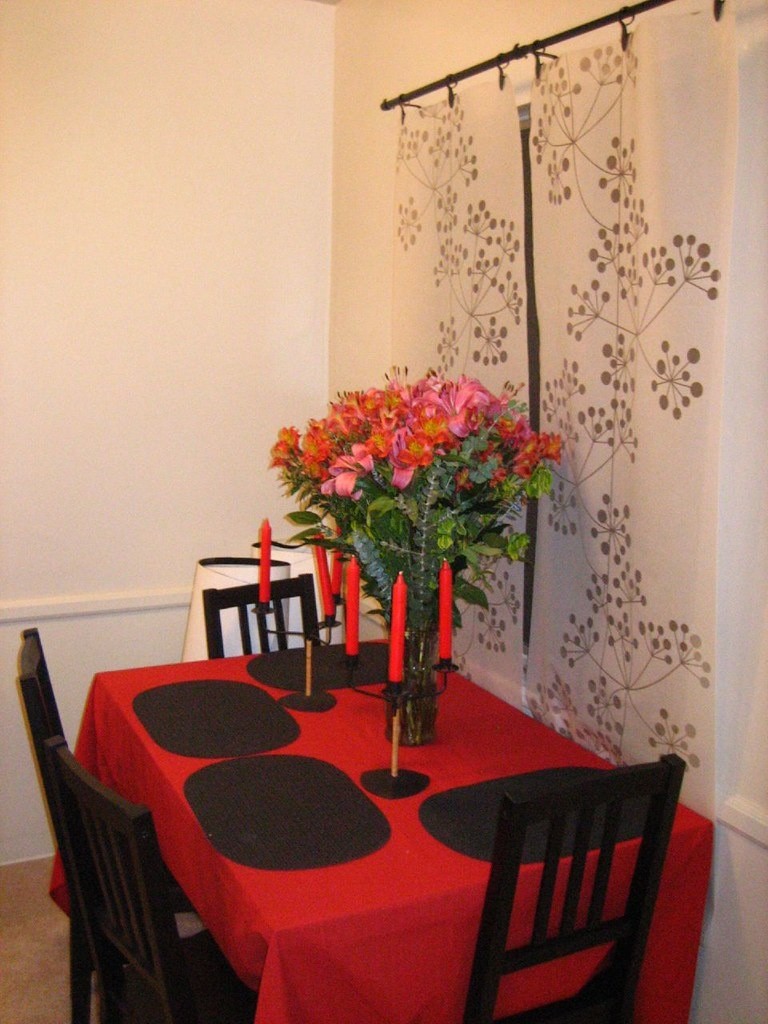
[269,364,566,625]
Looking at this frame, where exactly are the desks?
[50,639,715,1024]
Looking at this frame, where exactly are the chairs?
[202,574,322,660]
[41,737,263,1024]
[462,753,686,1024]
[15,628,67,776]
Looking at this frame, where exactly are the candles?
[388,569,404,681]
[310,528,333,617]
[332,522,342,594]
[256,517,270,601]
[437,555,453,661]
[343,555,362,654]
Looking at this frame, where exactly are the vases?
[376,597,438,745]
